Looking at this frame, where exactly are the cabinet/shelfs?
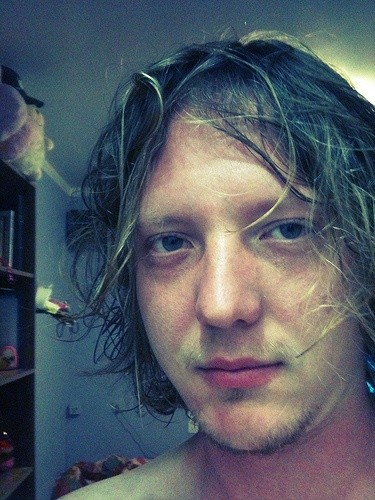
[0,156,38,500]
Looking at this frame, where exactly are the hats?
[0,64,45,109]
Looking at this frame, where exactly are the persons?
[47,30,375,500]
